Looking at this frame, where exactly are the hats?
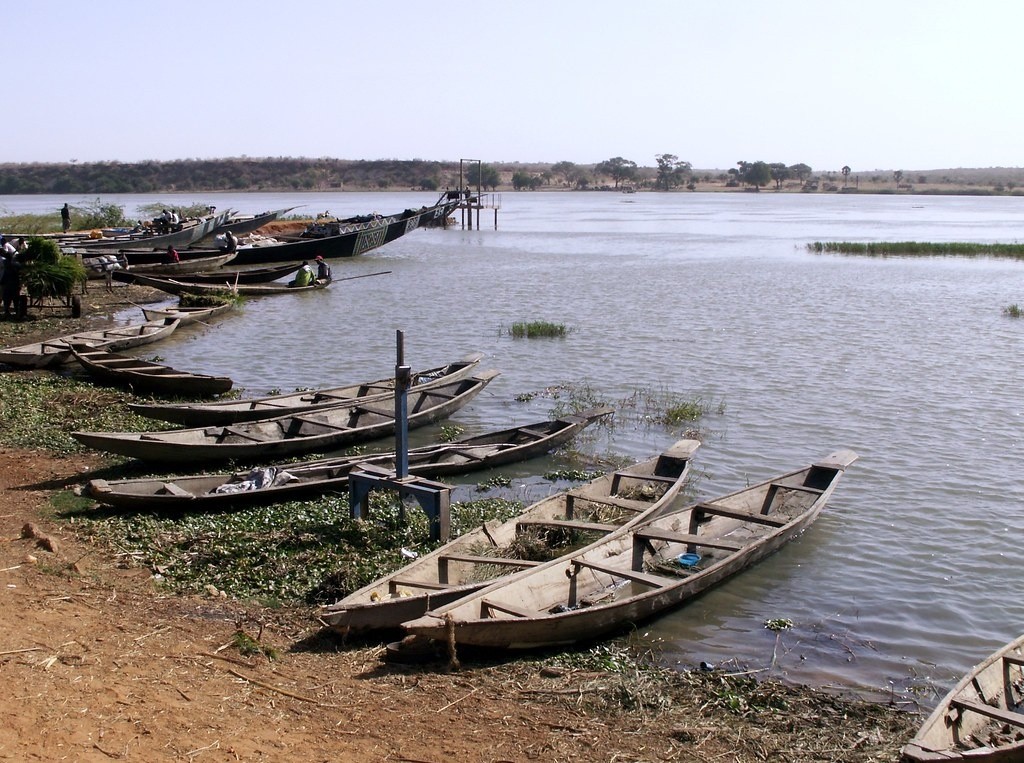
[315,256,323,261]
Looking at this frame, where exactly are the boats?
[71,404,615,508]
[896,637,1024,763]
[130,273,333,295]
[0,313,180,367]
[399,439,858,648]
[95,253,238,274]
[1,205,311,251]
[62,338,234,401]
[125,351,486,429]
[107,263,305,285]
[65,193,491,263]
[129,272,240,326]
[306,437,700,632]
[70,368,501,464]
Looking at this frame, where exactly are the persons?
[61,203,71,233]
[165,245,179,263]
[315,256,331,279]
[288,261,316,287]
[0,237,28,259]
[218,230,238,256]
[153,209,180,225]
[134,220,142,233]
[117,252,128,269]
[465,186,470,202]
